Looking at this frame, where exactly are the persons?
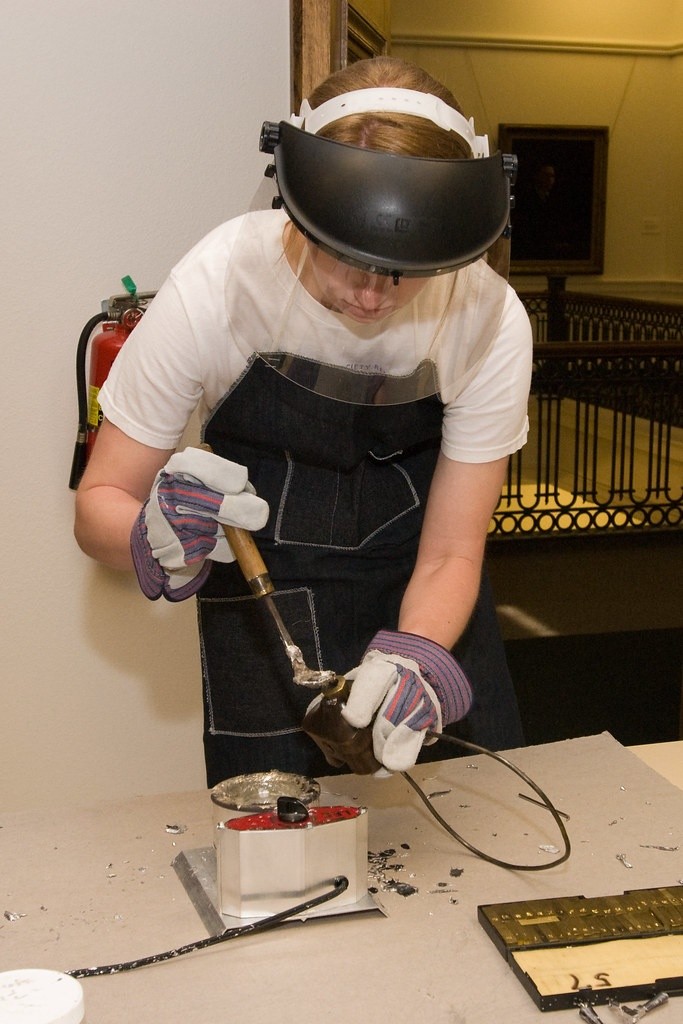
[70,57,535,788]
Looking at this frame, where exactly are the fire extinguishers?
[69,276,158,489]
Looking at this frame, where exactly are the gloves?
[340,628,475,773]
[126,447,272,603]
